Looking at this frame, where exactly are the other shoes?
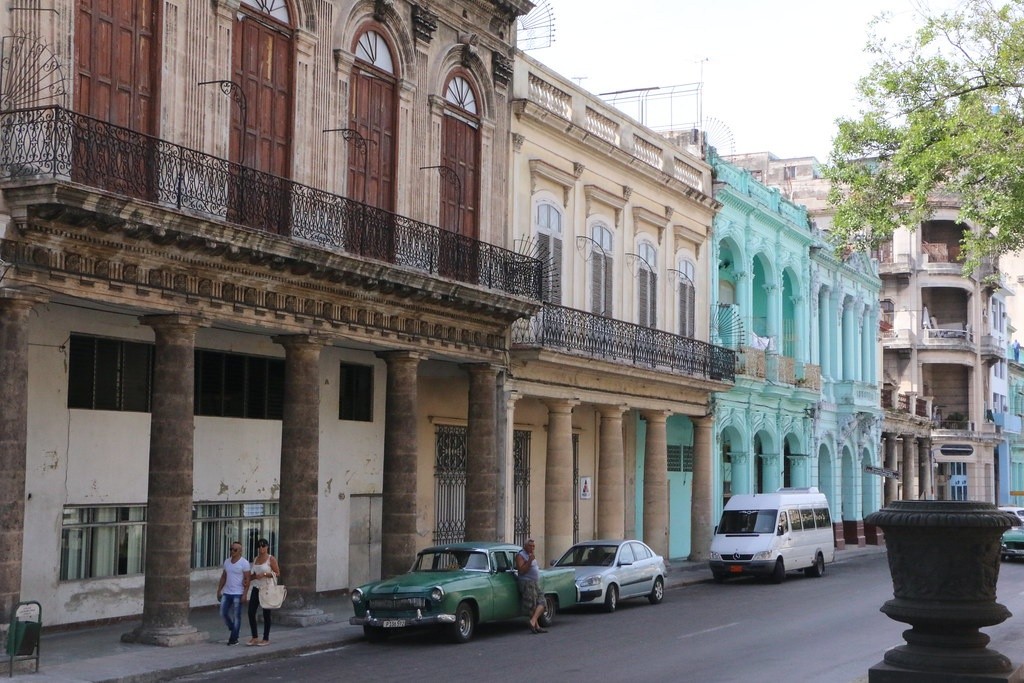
[227,635,239,647]
[527,621,548,634]
[246,638,269,646]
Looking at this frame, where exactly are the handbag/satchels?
[259,572,287,609]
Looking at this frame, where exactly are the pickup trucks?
[349,541,579,644]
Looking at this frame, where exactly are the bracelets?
[263,571,266,576]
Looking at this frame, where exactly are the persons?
[242,539,280,646]
[582,480,589,493]
[515,538,549,633]
[1013,340,1020,362]
[217,542,251,646]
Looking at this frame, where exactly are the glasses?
[230,548,237,552]
[257,544,267,548]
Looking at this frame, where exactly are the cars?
[550,539,669,613]
[1001,520,1024,563]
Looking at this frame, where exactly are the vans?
[709,486,834,585]
[998,507,1024,522]
